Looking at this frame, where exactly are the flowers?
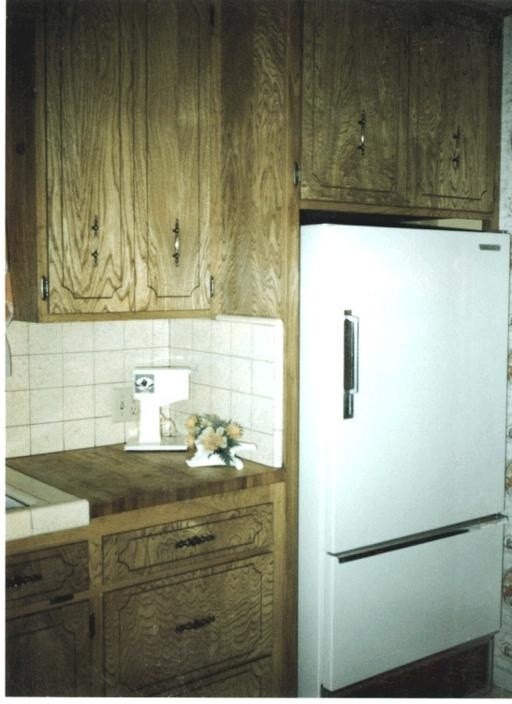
[184,414,241,449]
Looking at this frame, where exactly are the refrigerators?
[299,224,510,696]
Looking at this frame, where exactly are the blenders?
[122,364,194,452]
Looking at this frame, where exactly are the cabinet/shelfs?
[217,1,302,319]
[293,1,504,220]
[6,0,216,321]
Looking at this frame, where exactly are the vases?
[186,440,254,470]
